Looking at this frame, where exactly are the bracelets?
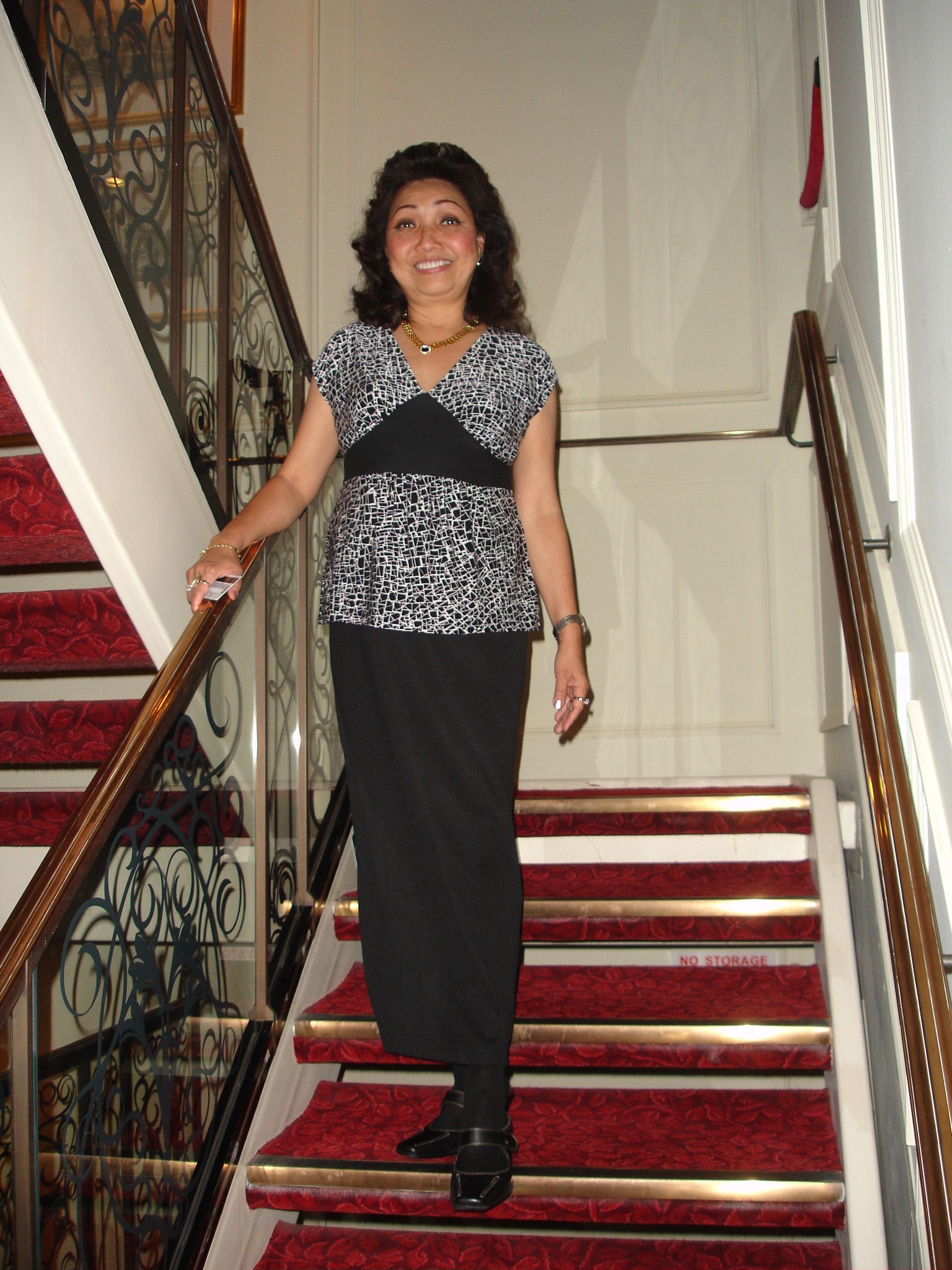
[201,543,241,560]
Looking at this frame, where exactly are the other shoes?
[450,1116,520,1213]
[396,1086,467,1159]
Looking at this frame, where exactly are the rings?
[199,579,210,588]
[569,699,573,702]
[185,584,191,591]
[191,573,202,588]
[574,696,590,705]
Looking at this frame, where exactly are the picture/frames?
[39,0,246,323]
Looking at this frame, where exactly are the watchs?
[552,612,587,642]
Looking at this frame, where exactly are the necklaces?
[401,306,481,355]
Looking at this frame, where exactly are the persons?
[184,141,596,1213]
